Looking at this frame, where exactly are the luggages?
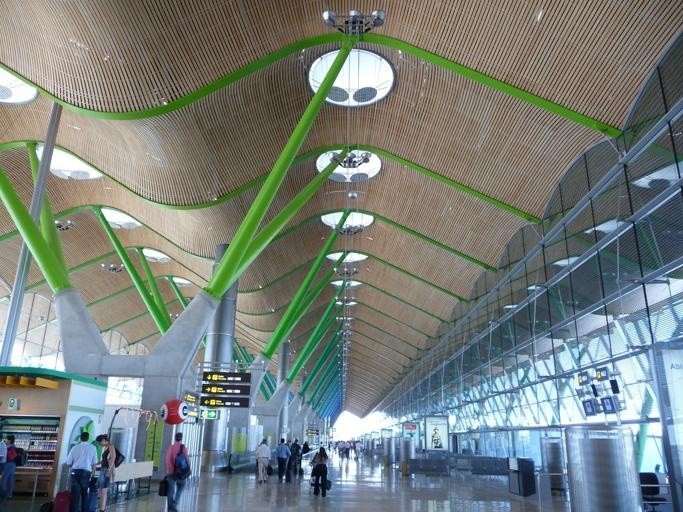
[54,490,71,512]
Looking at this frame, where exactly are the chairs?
[638,471,668,511]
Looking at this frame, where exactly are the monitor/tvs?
[582,399,601,416]
[601,395,622,414]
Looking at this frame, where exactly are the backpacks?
[174,444,192,481]
[15,447,27,467]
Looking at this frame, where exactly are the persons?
[255,436,310,485]
[166,432,191,512]
[431,426,443,449]
[330,440,363,458]
[0,430,8,478]
[310,446,329,498]
[1,434,17,501]
[96,433,116,512]
[67,432,97,512]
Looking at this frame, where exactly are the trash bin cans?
[506,457,536,497]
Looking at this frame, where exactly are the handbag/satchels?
[39,501,54,512]
[159,475,168,497]
[102,447,126,468]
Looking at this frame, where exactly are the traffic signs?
[186,393,200,418]
[199,371,251,409]
[306,429,318,436]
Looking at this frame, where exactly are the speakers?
[609,379,620,394]
[591,384,599,397]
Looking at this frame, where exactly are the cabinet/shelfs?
[0,429,58,496]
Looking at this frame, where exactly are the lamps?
[100,262,126,274]
[320,8,385,38]
[329,150,373,236]
[54,218,76,232]
